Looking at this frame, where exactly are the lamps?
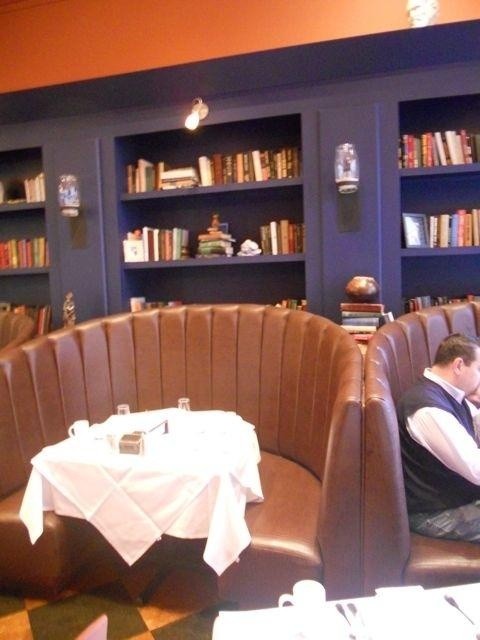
[185,96,209,130]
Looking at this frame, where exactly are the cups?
[68,396,190,455]
[277,578,331,640]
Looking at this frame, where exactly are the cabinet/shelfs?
[1,92,480,339]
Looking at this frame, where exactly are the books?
[120,146,307,263]
[127,295,308,315]
[401,291,480,315]
[0,168,50,272]
[335,302,396,344]
[427,207,480,249]
[1,301,52,339]
[396,128,480,173]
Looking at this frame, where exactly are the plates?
[265,608,350,640]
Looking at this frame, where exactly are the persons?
[394,332,480,549]
[61,289,77,330]
